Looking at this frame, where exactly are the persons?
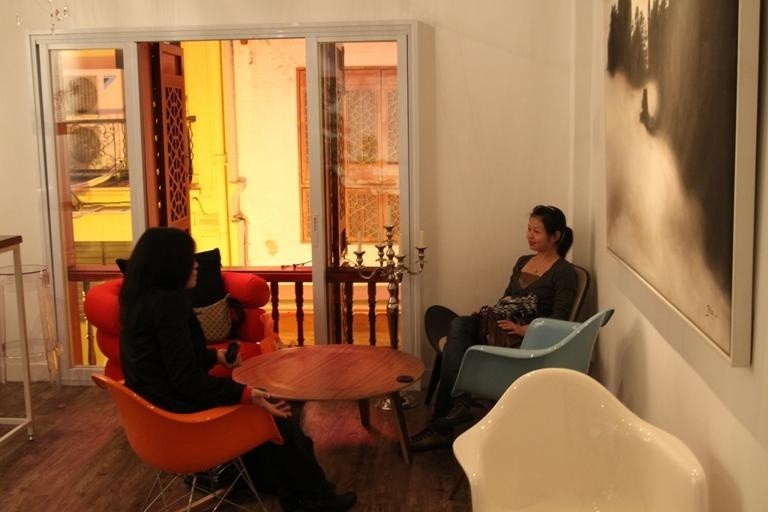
[119,227,357,512]
[394,205,581,452]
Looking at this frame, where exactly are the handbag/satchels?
[194,294,245,343]
[480,305,522,348]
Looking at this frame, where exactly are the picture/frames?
[605,1,759,367]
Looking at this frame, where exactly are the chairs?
[91,372,283,512]
[454,367,709,511]
[427,261,591,418]
[451,309,614,499]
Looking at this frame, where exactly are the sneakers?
[441,402,471,425]
[399,425,454,451]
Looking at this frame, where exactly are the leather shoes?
[310,491,358,512]
[279,483,336,511]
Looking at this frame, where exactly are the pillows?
[114,249,244,342]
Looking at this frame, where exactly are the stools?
[4,265,67,409]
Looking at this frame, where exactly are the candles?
[419,227,424,246]
[377,226,381,244]
[398,233,402,255]
[357,229,362,251]
[386,202,391,226]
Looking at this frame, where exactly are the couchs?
[84,270,283,382]
